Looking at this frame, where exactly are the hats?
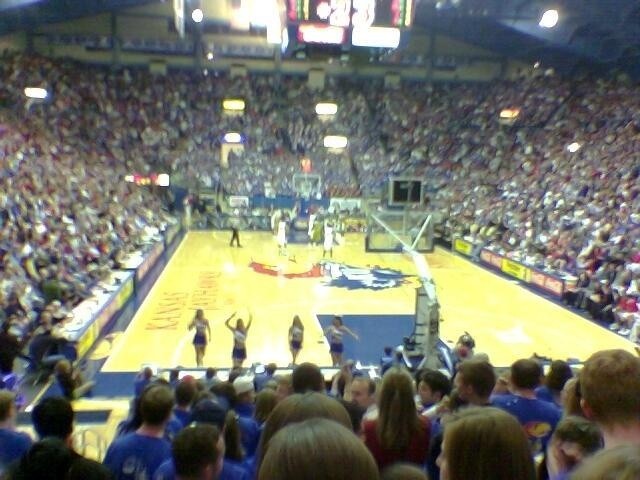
[190,398,228,427]
[232,376,255,397]
